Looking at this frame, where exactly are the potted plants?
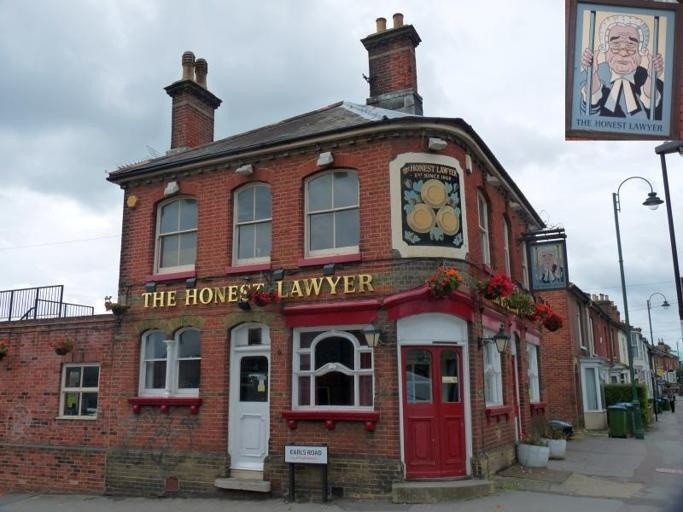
[106,301,129,316]
[516,425,574,468]
[48,338,72,355]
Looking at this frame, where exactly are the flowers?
[425,263,462,298]
[527,300,566,332]
[243,284,280,307]
[477,271,516,300]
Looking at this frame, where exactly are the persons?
[538,249,563,284]
[580,13,663,123]
[668,389,676,415]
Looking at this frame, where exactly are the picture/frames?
[526,239,569,292]
[562,0,682,146]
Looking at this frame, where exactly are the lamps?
[428,136,448,151]
[323,263,344,275]
[185,278,197,288]
[316,151,334,166]
[234,164,254,176]
[163,181,180,197]
[476,321,511,354]
[486,172,500,188]
[272,267,304,281]
[145,282,157,293]
[509,200,522,212]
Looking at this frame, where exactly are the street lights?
[647,292,670,396]
[613,176,663,439]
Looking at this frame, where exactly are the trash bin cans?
[662,397,671,410]
[608,403,634,438]
[656,400,661,413]
